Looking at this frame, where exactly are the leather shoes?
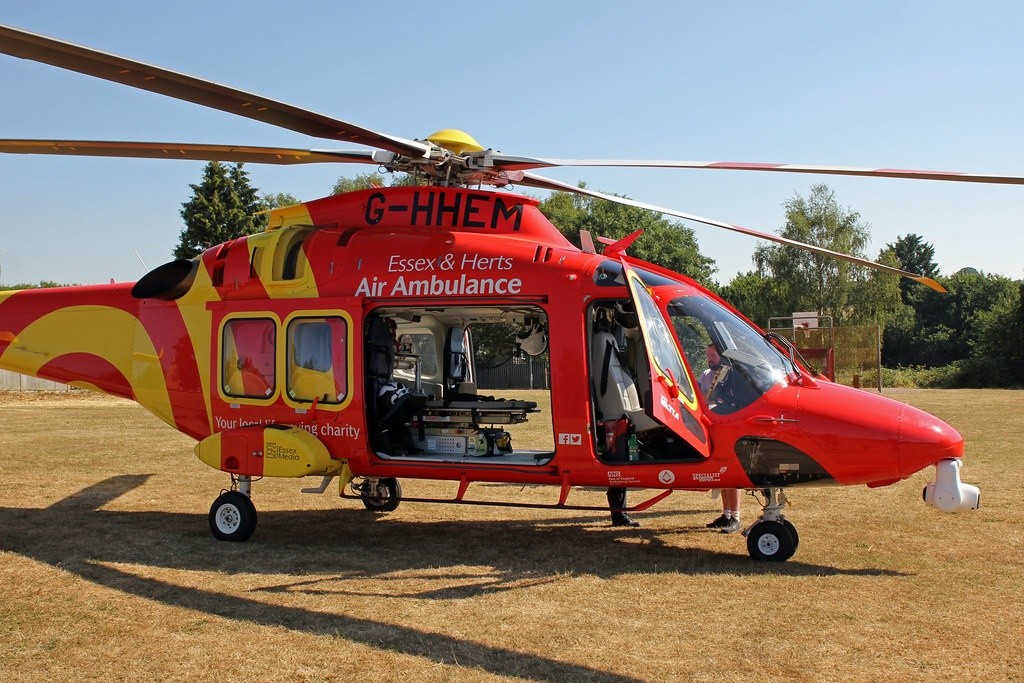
[613,515,640,526]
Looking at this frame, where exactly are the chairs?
[366,316,426,425]
[592,308,666,433]
[636,341,651,406]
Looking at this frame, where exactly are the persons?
[606,485,642,527]
[699,344,740,533]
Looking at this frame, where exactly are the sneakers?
[706,515,732,528]
[722,517,741,532]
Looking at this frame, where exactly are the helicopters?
[0,23,1024,563]
